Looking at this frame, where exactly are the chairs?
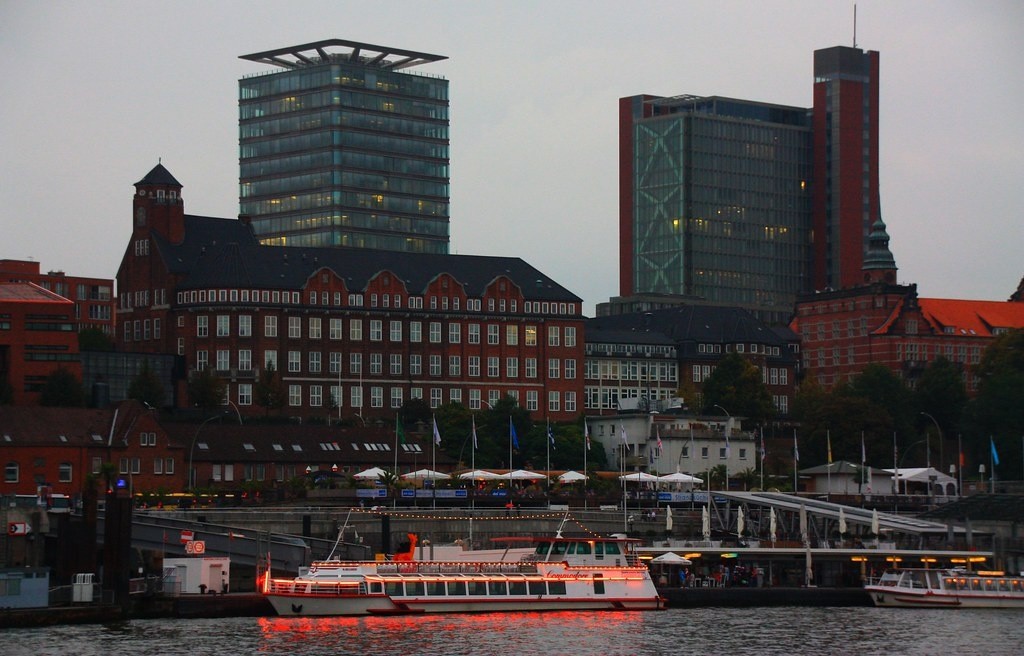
[720,579,726,588]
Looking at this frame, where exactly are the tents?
[882,468,957,496]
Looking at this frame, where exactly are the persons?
[647,557,778,588]
[701,523,888,545]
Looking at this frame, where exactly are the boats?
[863,566,1024,608]
[261,509,669,616]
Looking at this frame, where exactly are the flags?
[758,426,1005,467]
[656,429,663,453]
[474,418,477,450]
[433,417,442,446]
[620,424,632,452]
[511,423,518,447]
[585,419,591,451]
[689,429,696,453]
[724,430,731,456]
[548,421,557,451]
[397,417,406,442]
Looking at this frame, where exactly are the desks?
[694,578,701,587]
[708,578,715,587]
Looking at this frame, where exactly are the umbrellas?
[913,491,1024,521]
[649,552,692,587]
[965,515,973,548]
[353,468,703,490]
[666,502,880,542]
[805,544,813,587]
[946,517,953,547]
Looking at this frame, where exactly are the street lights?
[920,411,943,472]
[458,423,488,476]
[188,414,222,493]
[221,398,245,426]
[898,439,925,468]
